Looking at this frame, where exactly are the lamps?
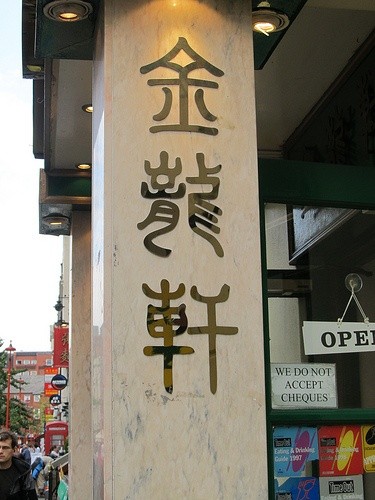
[54,295,69,311]
[52,4,86,21]
[252,14,281,34]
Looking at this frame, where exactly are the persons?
[0,431,61,500]
